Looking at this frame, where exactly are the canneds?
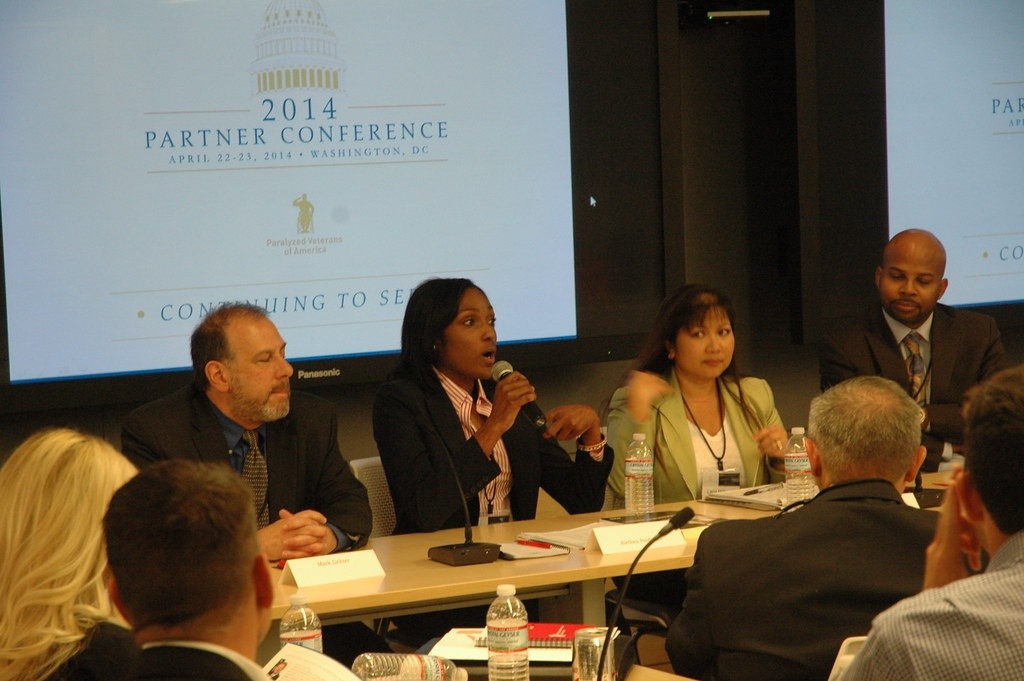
[571,628,616,681]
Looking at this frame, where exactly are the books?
[708,484,791,511]
[473,622,594,648]
[498,538,570,560]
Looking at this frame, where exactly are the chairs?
[607,590,679,637]
[350,457,396,536]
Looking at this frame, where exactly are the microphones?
[491,361,547,427]
[414,378,501,567]
[596,507,696,681]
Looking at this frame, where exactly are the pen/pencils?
[517,540,552,549]
[744,482,784,495]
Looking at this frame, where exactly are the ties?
[238,431,271,534]
[902,330,928,427]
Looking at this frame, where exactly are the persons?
[820,228,1012,472]
[1,429,140,680]
[840,365,1024,681]
[374,278,614,642]
[605,285,789,612]
[122,301,394,673]
[102,461,276,680]
[665,377,988,680]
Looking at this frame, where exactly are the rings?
[777,441,782,449]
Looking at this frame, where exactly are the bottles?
[278,593,323,654]
[486,584,529,681]
[350,652,468,681]
[784,426,815,509]
[624,433,655,513]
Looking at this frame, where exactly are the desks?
[269,472,951,627]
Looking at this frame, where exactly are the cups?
[575,628,614,681]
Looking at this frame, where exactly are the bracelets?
[576,433,608,452]
